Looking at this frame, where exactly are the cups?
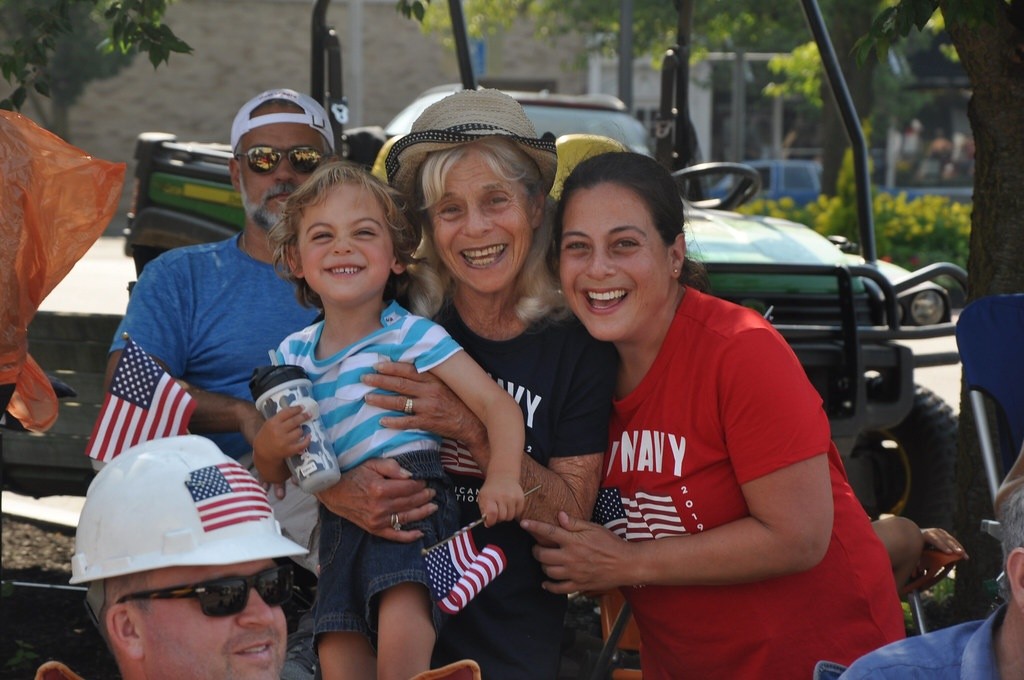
[248,365,341,495]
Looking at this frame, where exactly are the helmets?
[371,135,408,185]
[549,133,630,201]
[68,435,309,584]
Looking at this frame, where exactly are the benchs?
[0,313,914,680]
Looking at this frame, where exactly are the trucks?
[708,160,821,207]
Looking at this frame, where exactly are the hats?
[385,88,557,212]
[231,88,335,157]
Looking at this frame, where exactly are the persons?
[105,86,335,577]
[837,441,1024,680]
[312,89,617,680]
[901,119,954,178]
[251,163,524,680]
[70,433,310,680]
[519,150,904,679]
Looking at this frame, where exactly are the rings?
[405,397,413,414]
[391,514,401,531]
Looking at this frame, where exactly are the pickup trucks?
[122,93,966,542]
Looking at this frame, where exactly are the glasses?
[115,556,294,617]
[235,144,333,175]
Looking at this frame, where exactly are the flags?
[85,339,197,463]
[422,528,507,614]
